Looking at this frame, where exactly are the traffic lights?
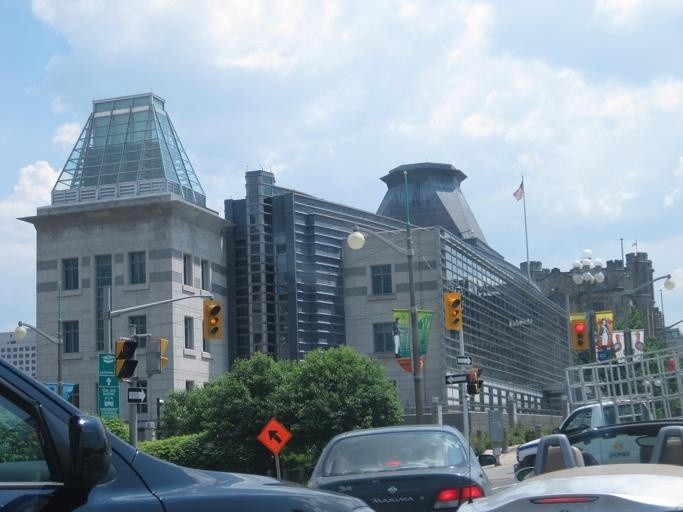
[146,338,169,375]
[444,292,462,331]
[203,300,224,339]
[115,340,139,380]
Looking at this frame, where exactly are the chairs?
[531,424,683,476]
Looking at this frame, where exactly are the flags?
[513,183,523,201]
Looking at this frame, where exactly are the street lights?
[572,238,677,362]
[15,281,63,397]
[347,170,423,425]
